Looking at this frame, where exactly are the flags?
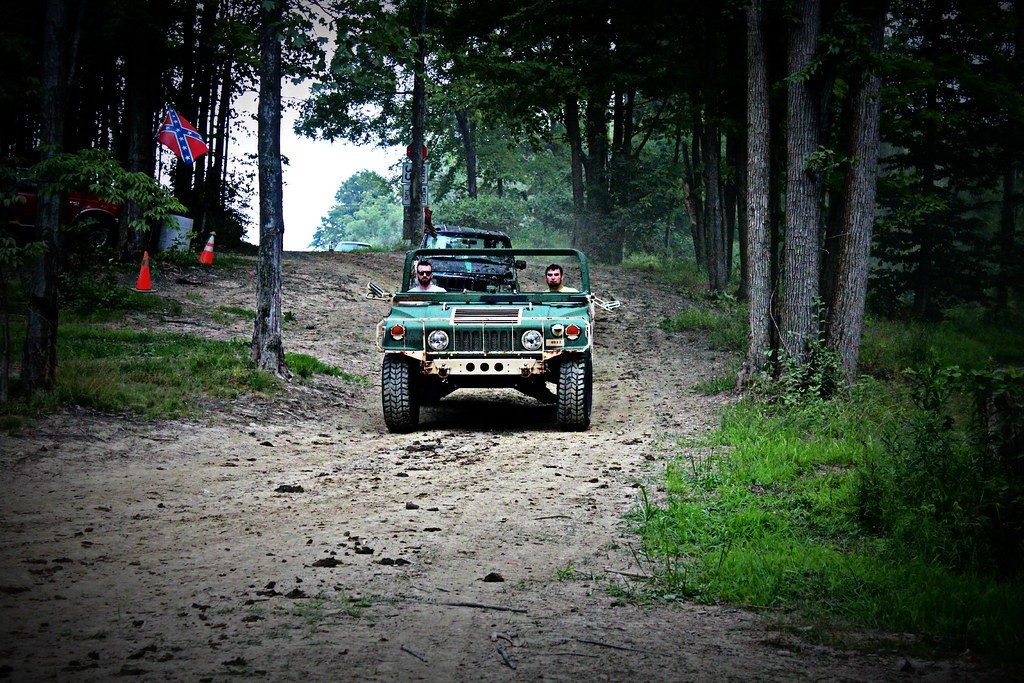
[157,105,210,166]
[424,207,437,239]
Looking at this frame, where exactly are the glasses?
[417,270,432,275]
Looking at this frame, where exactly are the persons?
[483,239,497,248]
[406,261,448,293]
[543,263,580,293]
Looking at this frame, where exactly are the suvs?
[411,225,526,293]
[0,165,122,250]
[375,246,594,433]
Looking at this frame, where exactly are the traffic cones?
[199,234,217,265]
[132,251,158,293]
